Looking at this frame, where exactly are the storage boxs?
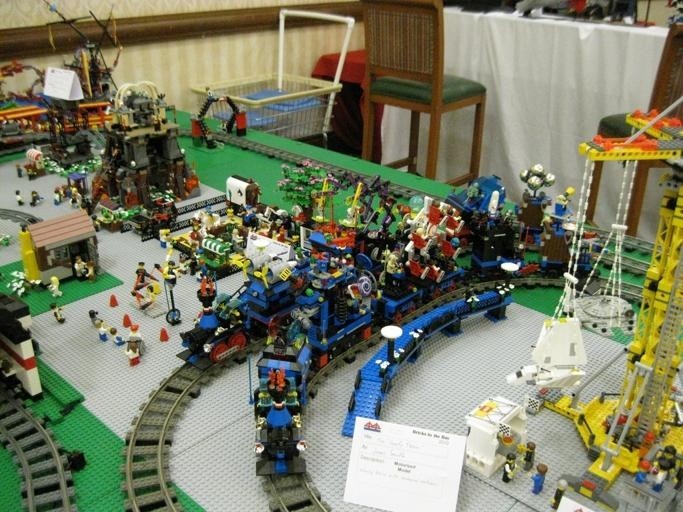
[214,89,321,140]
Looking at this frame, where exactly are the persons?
[395,200,464,243]
[227,203,255,244]
[651,445,678,489]
[502,441,568,510]
[14,164,197,347]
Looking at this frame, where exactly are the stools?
[312,50,383,165]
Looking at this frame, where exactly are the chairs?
[360,0,485,184]
[577,20,683,239]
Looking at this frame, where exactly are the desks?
[377,5,683,247]
